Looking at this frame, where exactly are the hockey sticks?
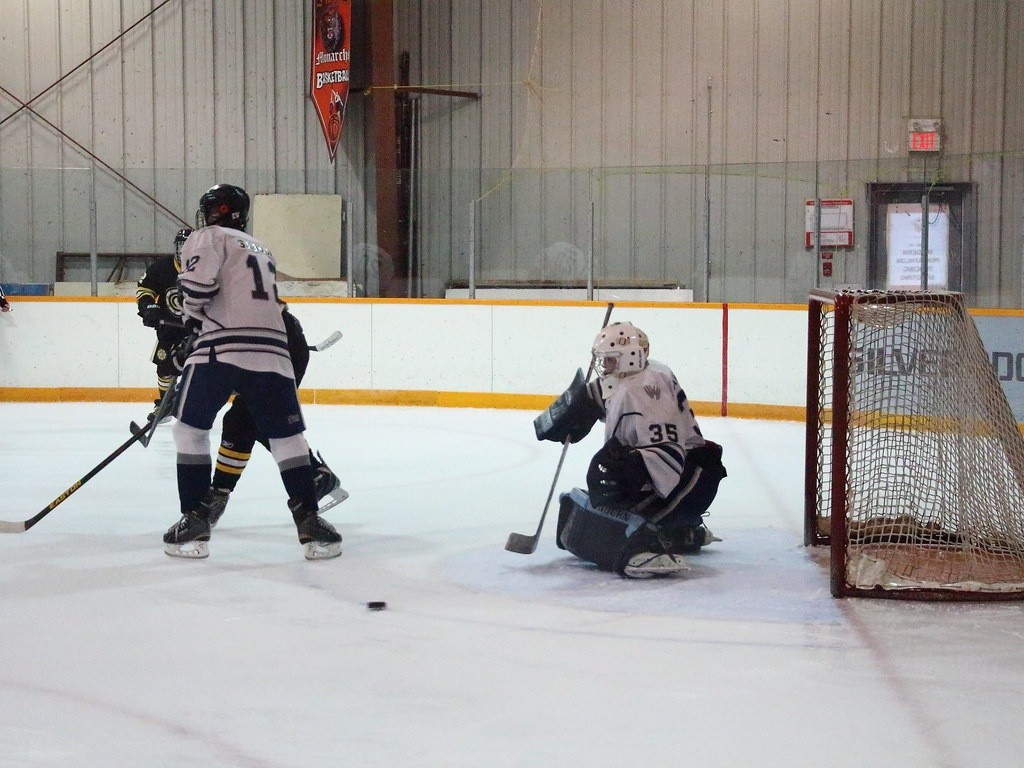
[504,303,616,554]
[130,336,193,448]
[160,318,344,351]
[0,401,173,534]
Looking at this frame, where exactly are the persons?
[534,321,727,580]
[0,285,10,312]
[136,184,350,561]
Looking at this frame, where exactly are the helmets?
[592,322,649,399]
[174,229,193,264]
[196,184,250,231]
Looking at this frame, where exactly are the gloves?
[142,305,161,328]
[164,343,187,375]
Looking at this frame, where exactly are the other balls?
[367,601,387,612]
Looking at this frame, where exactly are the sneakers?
[314,450,349,515]
[624,540,692,579]
[210,484,230,529]
[147,399,172,424]
[288,493,343,560]
[681,526,722,551]
[163,496,210,558]
